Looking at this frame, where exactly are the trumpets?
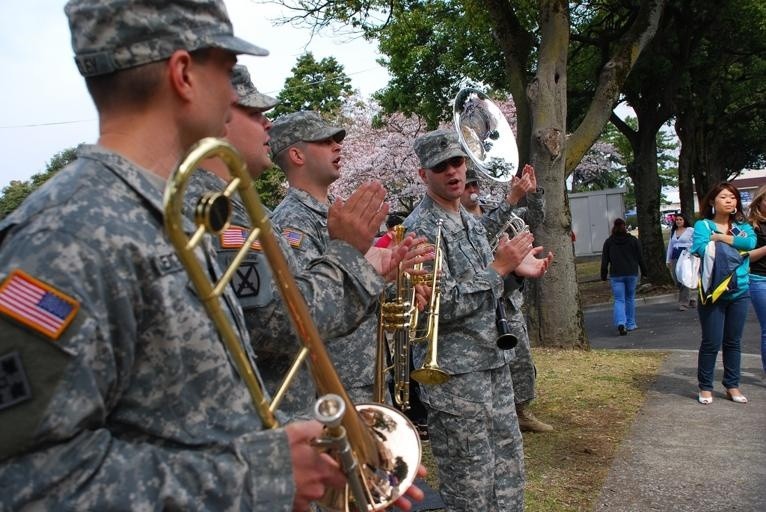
[409,218,450,385]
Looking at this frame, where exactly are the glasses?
[422,156,466,174]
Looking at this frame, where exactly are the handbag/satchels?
[674,220,710,290]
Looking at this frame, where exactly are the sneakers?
[679,298,697,311]
[617,321,639,335]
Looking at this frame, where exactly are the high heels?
[698,388,714,404]
[724,388,748,403]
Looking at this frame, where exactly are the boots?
[516,400,554,433]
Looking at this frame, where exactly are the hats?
[230,63,282,114]
[63,0,270,80]
[412,127,469,167]
[267,108,347,163]
[464,169,481,185]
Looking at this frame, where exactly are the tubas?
[453,87,532,263]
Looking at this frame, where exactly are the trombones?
[162,136,423,511]
[374,225,426,412]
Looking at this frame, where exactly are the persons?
[666,181,766,405]
[601,218,647,336]
